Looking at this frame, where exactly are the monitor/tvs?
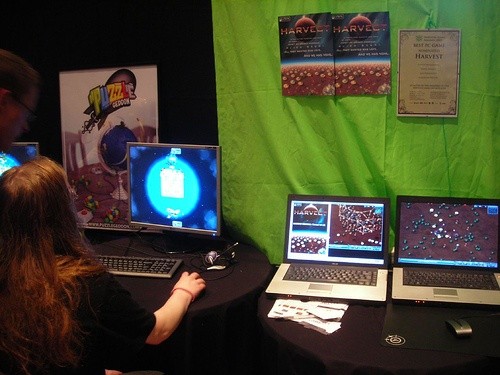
[126,142,222,236]
[0,142,40,177]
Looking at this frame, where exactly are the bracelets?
[171,287,195,302]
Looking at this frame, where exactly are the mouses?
[445,318,473,339]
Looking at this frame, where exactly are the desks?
[258,271,500,375]
[56,227,274,317]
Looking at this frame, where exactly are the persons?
[0,156,207,374]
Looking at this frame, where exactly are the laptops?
[264,193,391,307]
[391,194,500,309]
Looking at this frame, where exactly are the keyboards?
[92,255,183,279]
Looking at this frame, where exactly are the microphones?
[204,242,239,265]
